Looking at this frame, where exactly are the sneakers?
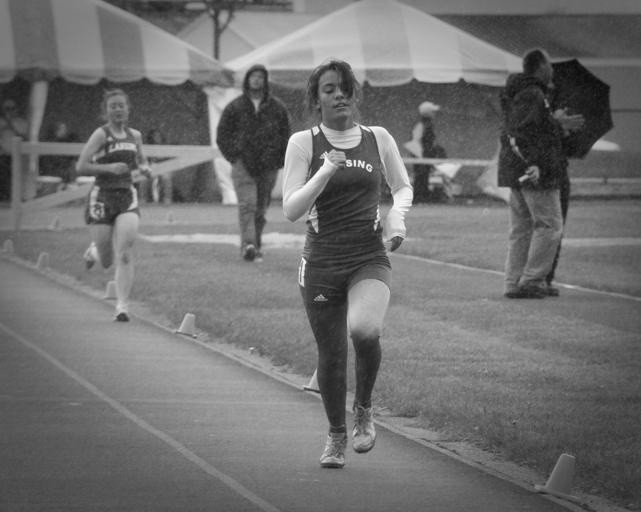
[319,423,345,467]
[113,301,127,319]
[82,240,98,268]
[242,244,263,265]
[351,404,377,453]
[505,284,560,299]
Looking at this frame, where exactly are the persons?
[0,98,29,201]
[45,122,73,183]
[497,48,563,298]
[284,59,415,467]
[216,64,293,263]
[76,88,150,322]
[412,101,441,203]
[543,80,572,297]
[147,128,174,205]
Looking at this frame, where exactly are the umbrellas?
[546,57,615,164]
[222,0,524,90]
[0,0,234,87]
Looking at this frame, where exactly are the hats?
[418,99,442,113]
